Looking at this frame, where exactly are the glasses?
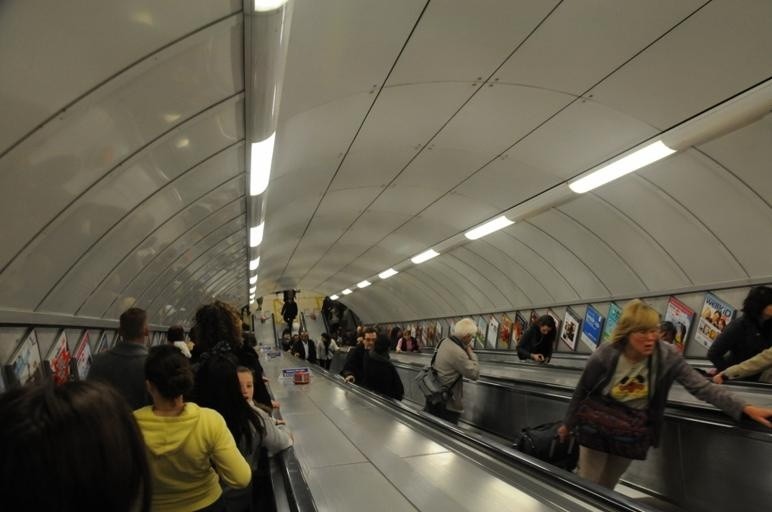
[362,336,378,343]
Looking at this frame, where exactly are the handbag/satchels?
[412,364,455,410]
[570,386,655,462]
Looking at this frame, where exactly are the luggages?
[513,418,582,477]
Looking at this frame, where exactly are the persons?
[660,321,676,341]
[416,318,479,422]
[701,324,717,338]
[703,306,711,321]
[274,291,405,402]
[517,316,557,364]
[712,347,772,386]
[716,319,726,330]
[1,291,295,512]
[708,310,721,326]
[557,299,772,490]
[706,285,771,372]
